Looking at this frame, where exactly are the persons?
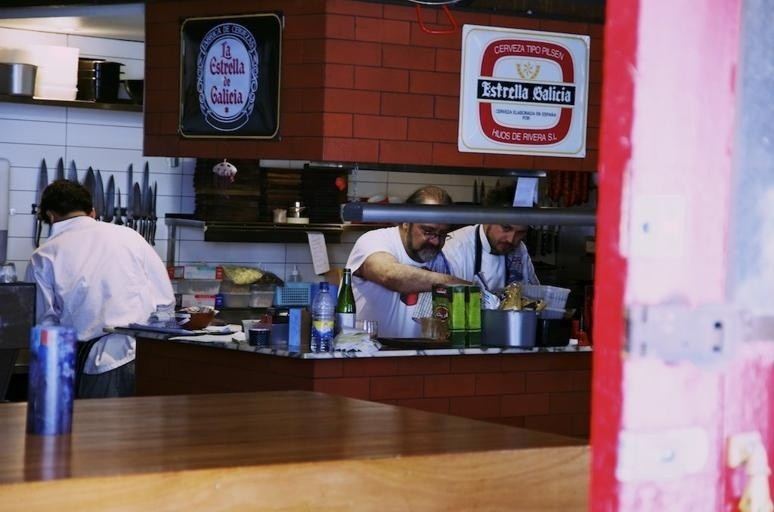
[441,182,541,292]
[22,178,177,399]
[336,185,474,339]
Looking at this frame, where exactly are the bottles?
[289,264,301,284]
[309,269,355,353]
[215,292,224,310]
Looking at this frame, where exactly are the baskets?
[273,281,311,308]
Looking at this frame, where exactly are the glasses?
[424,231,451,241]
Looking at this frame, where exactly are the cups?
[356,320,378,337]
[418,317,453,348]
[250,329,270,346]
[241,319,259,341]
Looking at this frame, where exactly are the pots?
[77,56,143,103]
[0,62,36,97]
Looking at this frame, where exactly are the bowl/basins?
[482,308,540,349]
[175,310,213,331]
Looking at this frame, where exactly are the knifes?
[32,156,158,246]
